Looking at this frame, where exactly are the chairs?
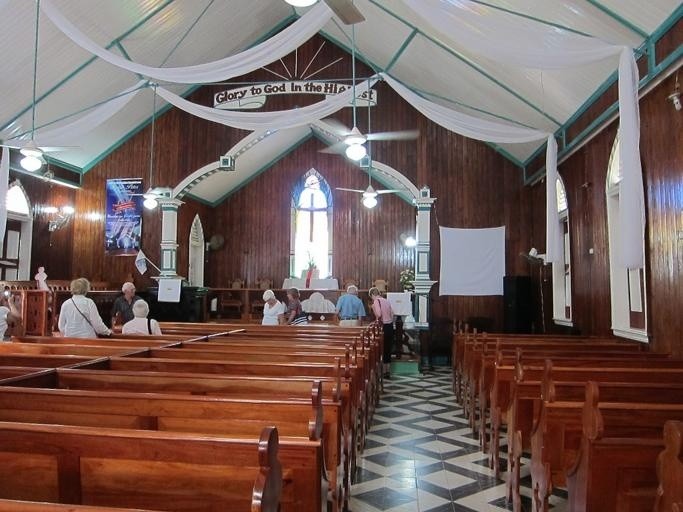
[217,275,274,319]
[337,278,389,299]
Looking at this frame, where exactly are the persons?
[57,278,112,339]
[110,282,143,329]
[335,285,366,326]
[285,288,308,325]
[261,290,285,326]
[122,299,163,336]
[368,287,394,377]
[0,287,20,342]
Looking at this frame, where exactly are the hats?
[263,290,274,301]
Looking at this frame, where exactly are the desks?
[201,288,371,321]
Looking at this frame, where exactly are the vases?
[403,281,413,290]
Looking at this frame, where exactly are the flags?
[135,251,147,275]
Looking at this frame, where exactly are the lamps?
[43,205,75,232]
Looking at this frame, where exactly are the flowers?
[400,269,415,282]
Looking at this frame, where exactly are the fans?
[305,2,419,162]
[2,2,82,171]
[519,247,548,268]
[334,69,406,210]
[122,81,186,209]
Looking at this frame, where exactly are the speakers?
[503,276,532,334]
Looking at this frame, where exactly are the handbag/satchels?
[369,320,383,327]
[286,312,308,326]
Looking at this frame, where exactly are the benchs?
[0,315,385,511]
[450,315,683,512]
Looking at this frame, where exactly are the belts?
[341,318,356,320]
